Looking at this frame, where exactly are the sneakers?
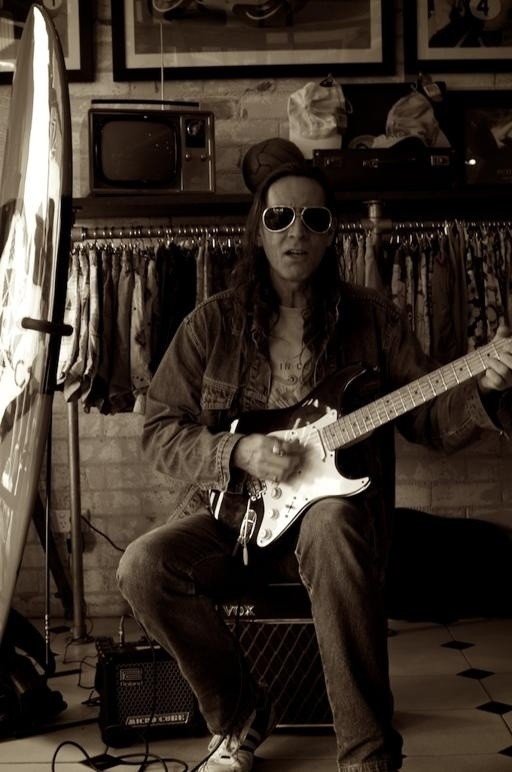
[203,703,276,771]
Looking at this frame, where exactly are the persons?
[117,135,511,772]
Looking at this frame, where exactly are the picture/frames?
[0,0,512,83]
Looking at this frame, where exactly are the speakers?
[94,641,208,746]
[214,581,334,735]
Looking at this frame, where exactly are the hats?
[372,91,452,149]
[287,76,348,161]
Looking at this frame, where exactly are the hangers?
[291,218,294,221]
[71,220,511,256]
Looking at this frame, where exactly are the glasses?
[262,204,332,234]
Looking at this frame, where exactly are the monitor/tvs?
[81,106,217,195]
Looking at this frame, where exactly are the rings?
[272,439,287,456]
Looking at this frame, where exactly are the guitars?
[207,336,509,568]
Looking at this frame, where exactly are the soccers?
[242,137,307,193]
